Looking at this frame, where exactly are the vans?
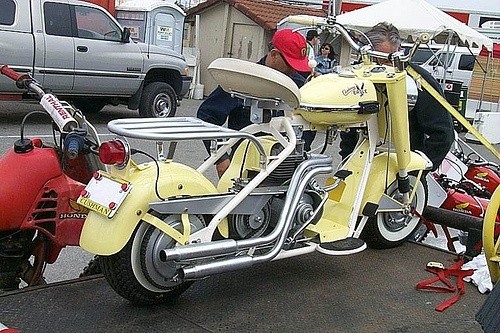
[0,1,193,118]
[408,49,480,98]
[397,43,456,64]
[390,43,482,98]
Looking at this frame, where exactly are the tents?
[331,0,493,114]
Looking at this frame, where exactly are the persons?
[314,43,339,76]
[305,30,319,69]
[195,28,316,179]
[338,19,456,174]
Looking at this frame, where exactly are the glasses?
[321,46,331,50]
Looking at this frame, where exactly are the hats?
[271,28,311,72]
[307,30,320,36]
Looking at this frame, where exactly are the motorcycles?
[442,128,500,199]
[76,15,433,305]
[413,171,500,254]
[0,63,112,294]
